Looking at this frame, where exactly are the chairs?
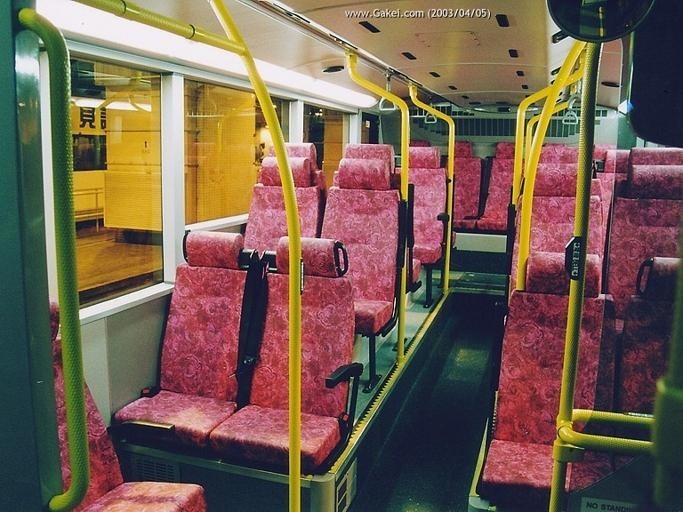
[111,136,482,511]
[46,303,205,512]
[473,142,682,512]
